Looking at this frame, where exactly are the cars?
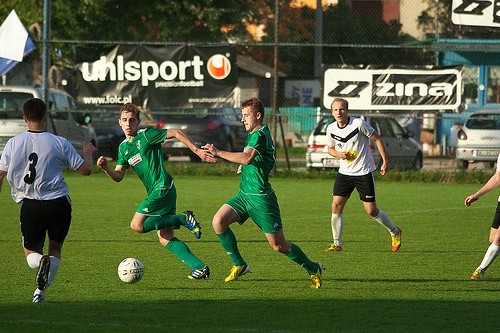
[147,102,250,163]
[83,104,160,161]
[456,108,500,169]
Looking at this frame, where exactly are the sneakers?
[32,294,45,303]
[35,255,51,291]
[308,262,323,290]
[179,265,211,282]
[390,226,403,252]
[181,210,203,239]
[324,243,343,252]
[224,265,250,283]
[470,270,485,280]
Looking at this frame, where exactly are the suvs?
[305,113,424,171]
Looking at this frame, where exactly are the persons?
[96,103,217,279]
[324,98,402,252]
[0,98,96,304]
[201,97,324,289]
[465,153,500,279]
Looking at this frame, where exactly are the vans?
[0,85,99,163]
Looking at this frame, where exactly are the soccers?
[117,257,145,284]
